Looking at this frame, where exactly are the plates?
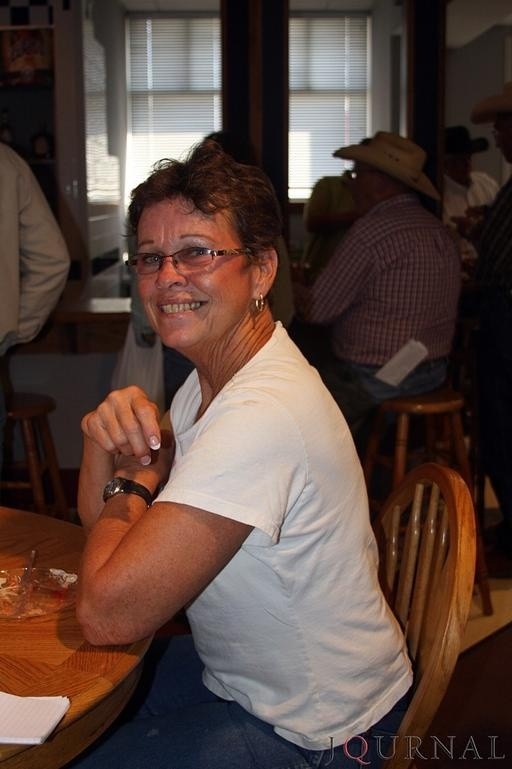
[0,568,80,619]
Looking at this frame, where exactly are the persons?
[76,144,415,769]
[2,129,71,512]
[112,78,512,569]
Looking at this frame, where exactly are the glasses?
[125,246,256,275]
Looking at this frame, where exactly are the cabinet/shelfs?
[0,1,91,259]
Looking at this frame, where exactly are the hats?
[469,81,512,125]
[332,130,442,202]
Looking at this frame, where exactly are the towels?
[0,691,70,744]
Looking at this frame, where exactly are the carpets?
[459,474,512,656]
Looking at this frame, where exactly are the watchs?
[98,475,157,514]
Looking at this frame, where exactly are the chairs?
[373,462,477,769]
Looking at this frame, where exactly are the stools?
[363,392,504,616]
[0,389,69,522]
